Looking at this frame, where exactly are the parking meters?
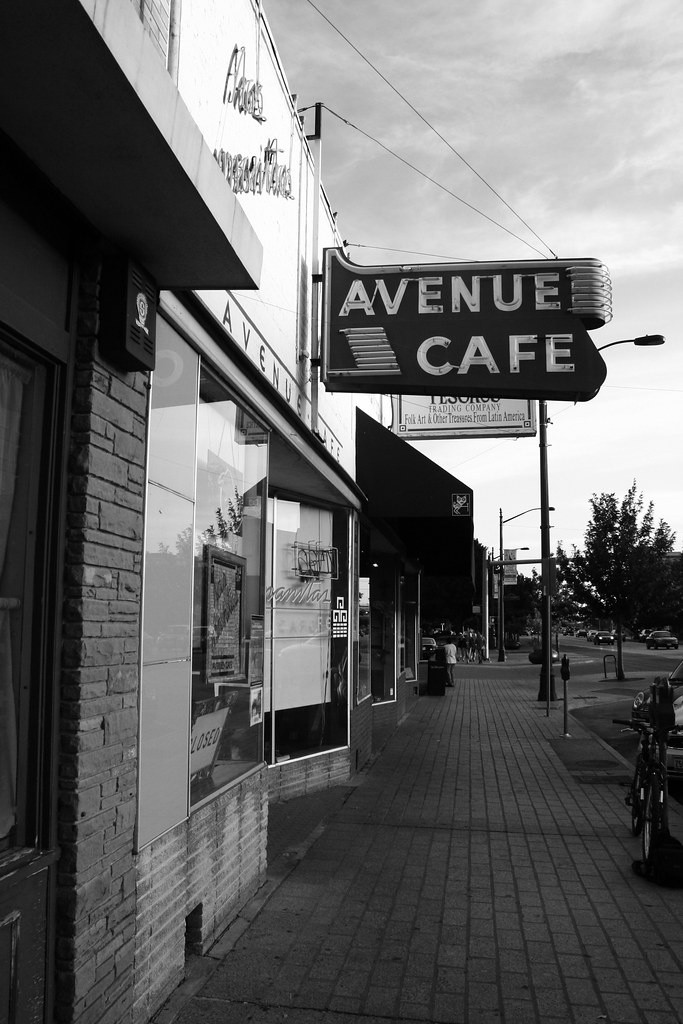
[560,653,572,739]
[648,676,675,810]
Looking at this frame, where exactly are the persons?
[458,634,490,665]
[443,639,458,686]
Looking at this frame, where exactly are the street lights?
[498,506,556,663]
[538,333,664,703]
[490,545,530,617]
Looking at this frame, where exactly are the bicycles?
[612,716,668,870]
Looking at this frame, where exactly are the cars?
[575,630,586,637]
[593,630,614,645]
[611,630,626,642]
[637,685,683,778]
[645,630,679,649]
[639,629,653,643]
[421,636,438,660]
[562,628,574,636]
[631,659,683,722]
[586,630,598,642]
